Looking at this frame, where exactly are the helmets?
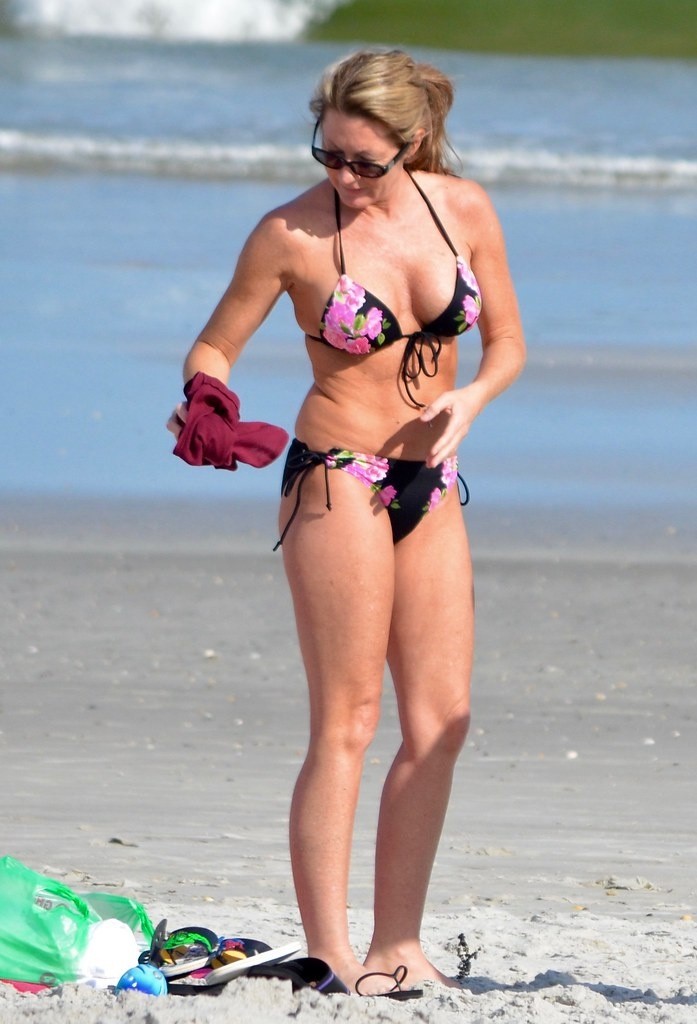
[116,964,166,997]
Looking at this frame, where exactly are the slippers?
[355,965,423,1000]
[157,926,224,977]
[167,983,228,997]
[246,956,352,997]
[137,918,167,967]
[205,938,303,987]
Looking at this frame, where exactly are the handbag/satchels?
[0,854,153,987]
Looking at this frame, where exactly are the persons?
[164,45,528,999]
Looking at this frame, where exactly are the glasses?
[312,109,415,179]
